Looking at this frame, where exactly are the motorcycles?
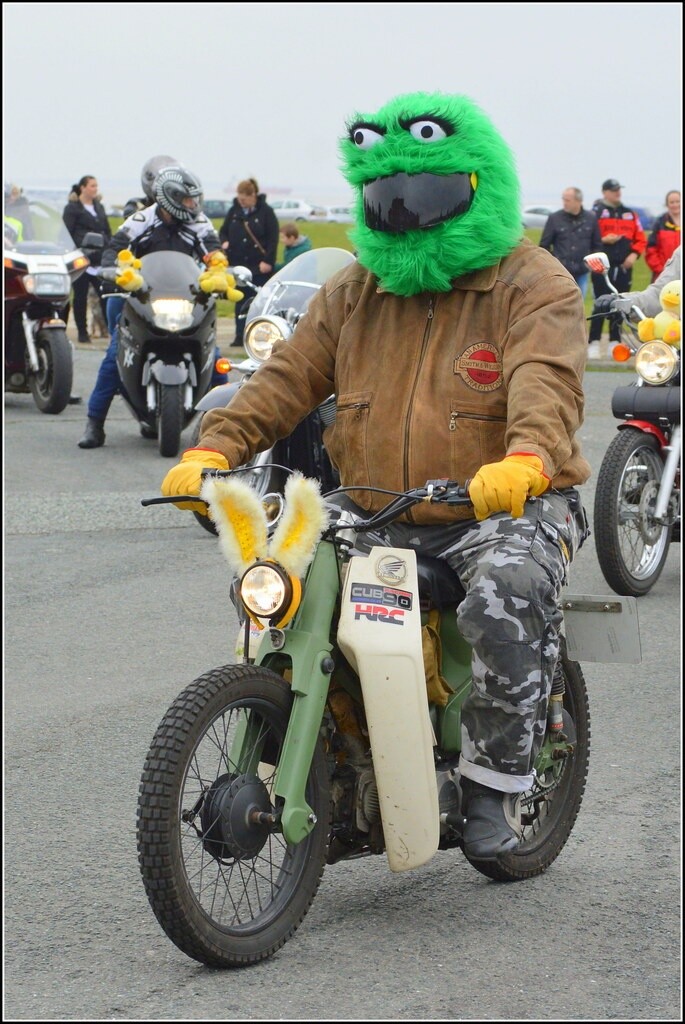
[186,246,358,535]
[3,199,106,413]
[580,250,681,597]
[99,250,229,455]
[138,464,591,968]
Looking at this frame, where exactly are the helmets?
[141,156,182,202]
[363,171,478,233]
[152,168,203,223]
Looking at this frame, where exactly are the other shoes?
[609,342,619,359]
[79,333,90,342]
[68,395,83,404]
[625,482,657,507]
[230,339,243,346]
[588,340,600,360]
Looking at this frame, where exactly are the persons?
[273,223,311,271]
[539,179,681,323]
[160,88,593,863]
[218,177,279,346]
[4,153,228,449]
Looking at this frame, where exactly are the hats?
[602,179,626,191]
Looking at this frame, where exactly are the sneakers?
[460,777,521,860]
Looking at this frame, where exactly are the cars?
[270,200,315,222]
[627,205,659,230]
[199,198,235,218]
[521,205,552,227]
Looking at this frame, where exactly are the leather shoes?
[77,420,106,448]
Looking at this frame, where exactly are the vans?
[326,204,355,224]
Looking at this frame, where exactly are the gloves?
[469,451,551,521]
[161,448,230,522]
[593,294,629,321]
